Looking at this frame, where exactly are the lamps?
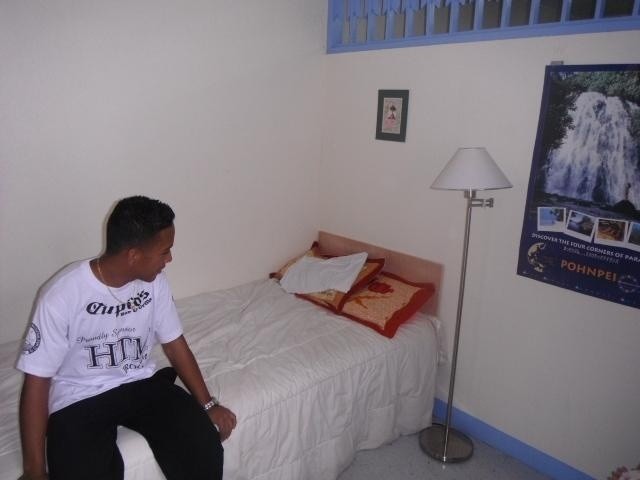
[418,145,515,465]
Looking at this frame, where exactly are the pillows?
[267,239,437,338]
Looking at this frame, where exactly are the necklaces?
[96,259,136,304]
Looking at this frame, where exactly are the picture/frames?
[375,89,410,142]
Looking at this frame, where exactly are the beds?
[0,228,447,480]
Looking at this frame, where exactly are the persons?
[14,196,237,480]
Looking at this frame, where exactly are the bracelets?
[204,396,219,411]
